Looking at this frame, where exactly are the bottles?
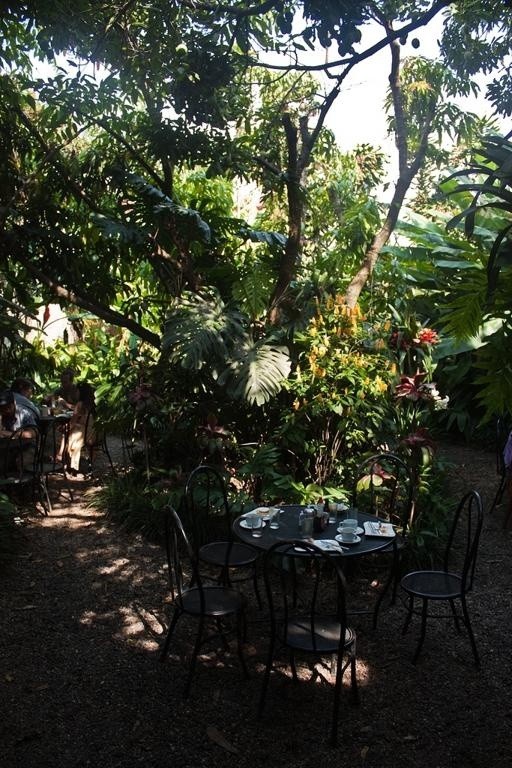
[299,496,328,527]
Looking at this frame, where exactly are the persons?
[503,430,511,470]
[0,371,97,476]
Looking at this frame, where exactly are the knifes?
[321,541,350,550]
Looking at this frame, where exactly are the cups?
[269,509,280,529]
[328,504,337,524]
[246,514,259,527]
[339,519,358,532]
[302,518,313,537]
[342,527,358,542]
[252,516,263,538]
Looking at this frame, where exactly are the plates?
[337,526,364,535]
[364,522,395,538]
[239,519,266,529]
[295,538,341,552]
[241,506,279,520]
[335,534,361,544]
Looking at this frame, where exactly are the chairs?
[165,504,253,696]
[1,396,114,517]
[254,539,359,737]
[488,417,511,530]
[400,489,483,663]
[153,451,414,653]
